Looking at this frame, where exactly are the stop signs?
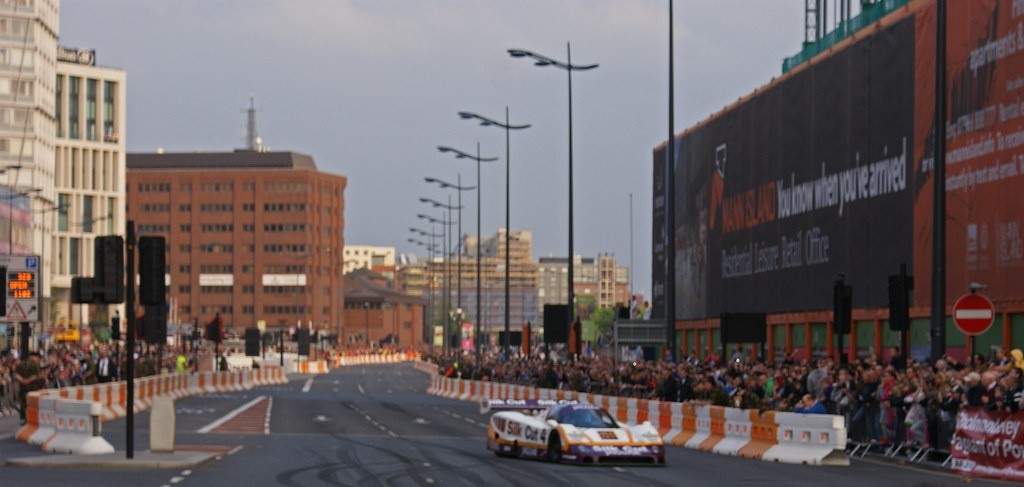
[953,293,994,337]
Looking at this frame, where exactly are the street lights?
[507,44,601,362]
[438,142,497,367]
[408,173,475,361]
[458,107,532,359]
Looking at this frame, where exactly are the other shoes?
[21,419,26,426]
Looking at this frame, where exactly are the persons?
[0,338,261,425]
[642,301,652,320]
[629,295,637,319]
[419,340,1023,462]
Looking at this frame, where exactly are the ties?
[100,360,105,376]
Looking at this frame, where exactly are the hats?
[30,352,41,358]
[963,372,980,383]
